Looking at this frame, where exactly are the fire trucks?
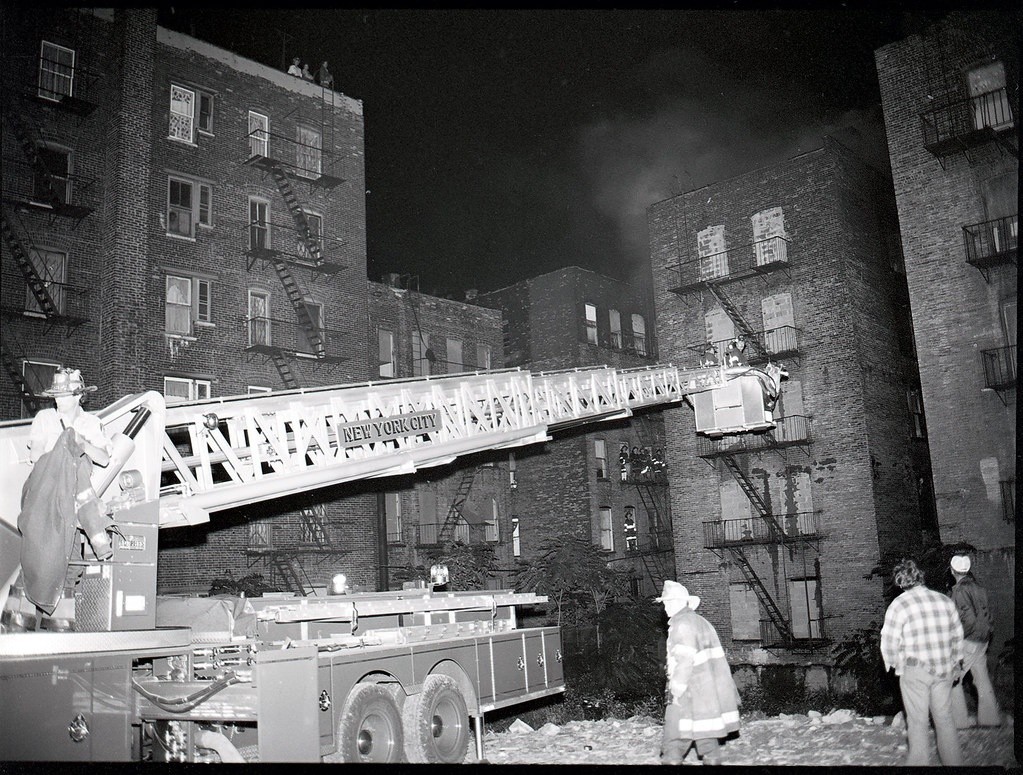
[0,341,790,761]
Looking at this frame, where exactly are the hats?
[951,555,970,573]
[41,368,98,398]
[653,579,700,610]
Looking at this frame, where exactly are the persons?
[653,579,742,765]
[25,367,114,634]
[619,445,668,484]
[950,554,1002,728]
[879,558,965,766]
[699,341,748,370]
[623,511,638,551]
[286,57,335,89]
